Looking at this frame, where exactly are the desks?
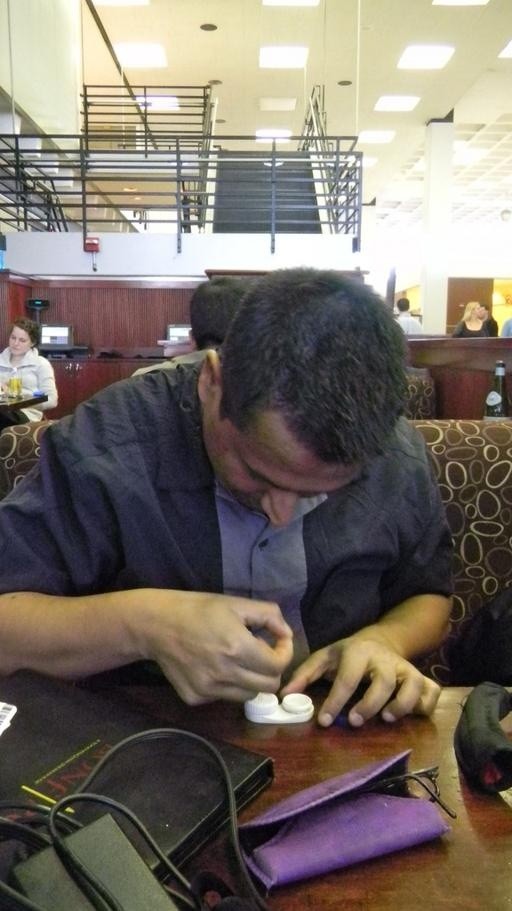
[89,678,512,911]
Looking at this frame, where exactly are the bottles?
[482,358,511,422]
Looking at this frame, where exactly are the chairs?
[2,416,511,685]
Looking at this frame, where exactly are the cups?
[8,376,21,398]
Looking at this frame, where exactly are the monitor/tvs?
[167,323,193,341]
[37,322,73,344]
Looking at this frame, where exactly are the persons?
[2,268,458,731]
[451,301,489,339]
[130,278,245,380]
[395,297,424,337]
[479,304,498,338]
[0,316,59,432]
